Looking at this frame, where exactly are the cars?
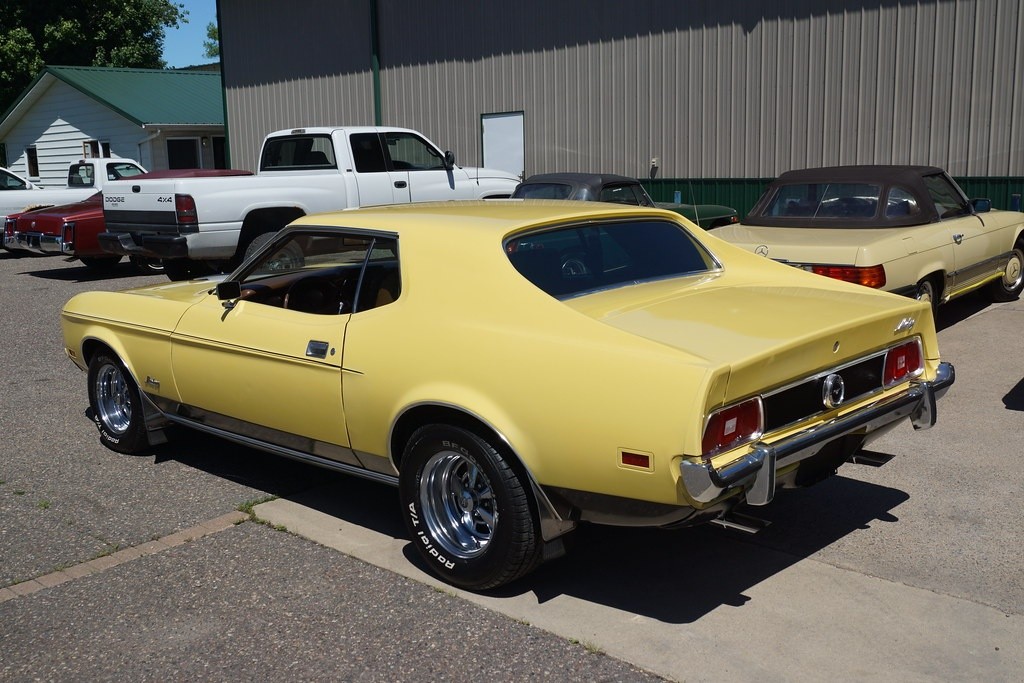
[514,173,738,230]
[5,170,256,268]
[707,164,1023,331]
[60,197,954,592]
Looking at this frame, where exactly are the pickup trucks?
[102,126,523,281]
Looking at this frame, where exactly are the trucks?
[0,158,149,244]
